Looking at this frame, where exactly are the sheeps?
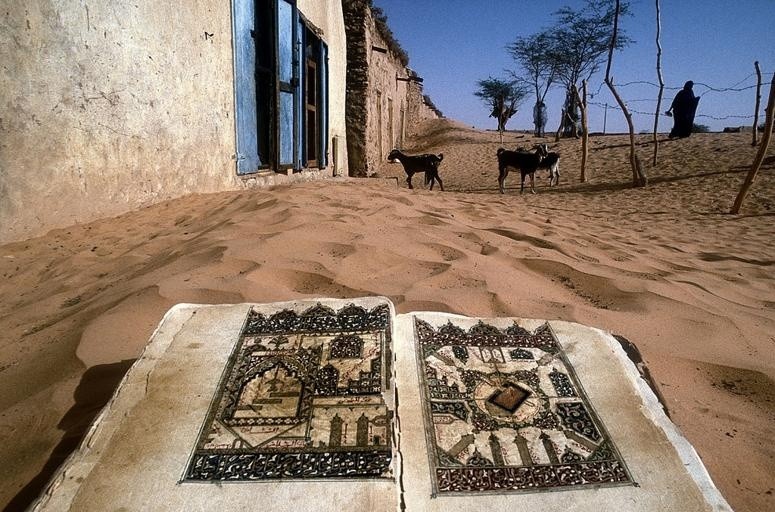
[515,146,562,186]
[387,148,446,193]
[495,143,548,192]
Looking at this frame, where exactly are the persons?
[668,81,700,138]
[533,98,547,137]
